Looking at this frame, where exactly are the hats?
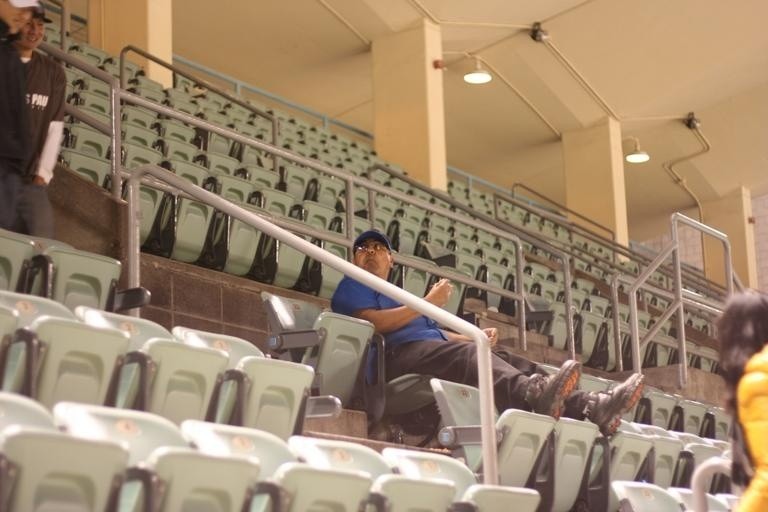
[30,8,53,23]
[9,0,42,9]
[353,229,392,254]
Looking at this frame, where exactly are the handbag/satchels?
[397,401,441,436]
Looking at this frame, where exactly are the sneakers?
[524,359,583,421]
[581,372,645,438]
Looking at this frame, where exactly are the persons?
[0,0,37,230]
[12,1,67,235]
[710,288,767,512]
[330,226,647,435]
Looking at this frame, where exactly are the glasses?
[354,242,386,252]
